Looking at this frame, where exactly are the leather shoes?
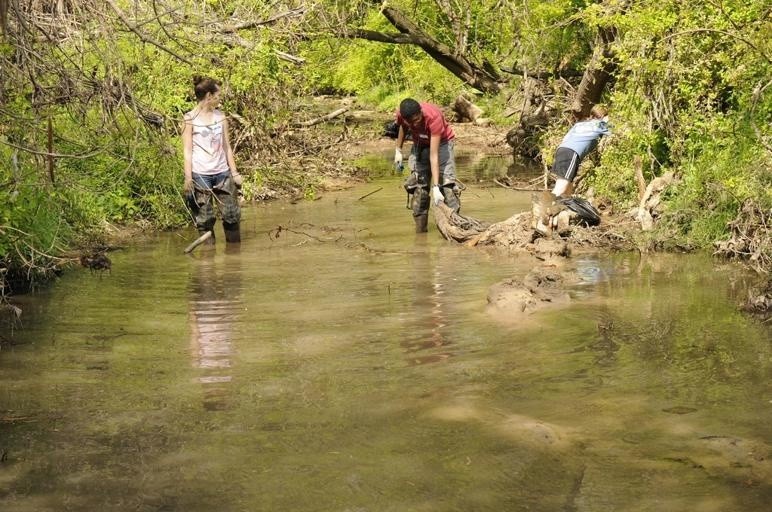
[233,172,242,189]
[394,148,403,169]
[433,186,444,205]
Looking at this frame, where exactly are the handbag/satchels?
[232,172,240,176]
[432,184,440,187]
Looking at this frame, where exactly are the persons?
[394,98,461,233]
[551,103,615,198]
[183,75,243,244]
